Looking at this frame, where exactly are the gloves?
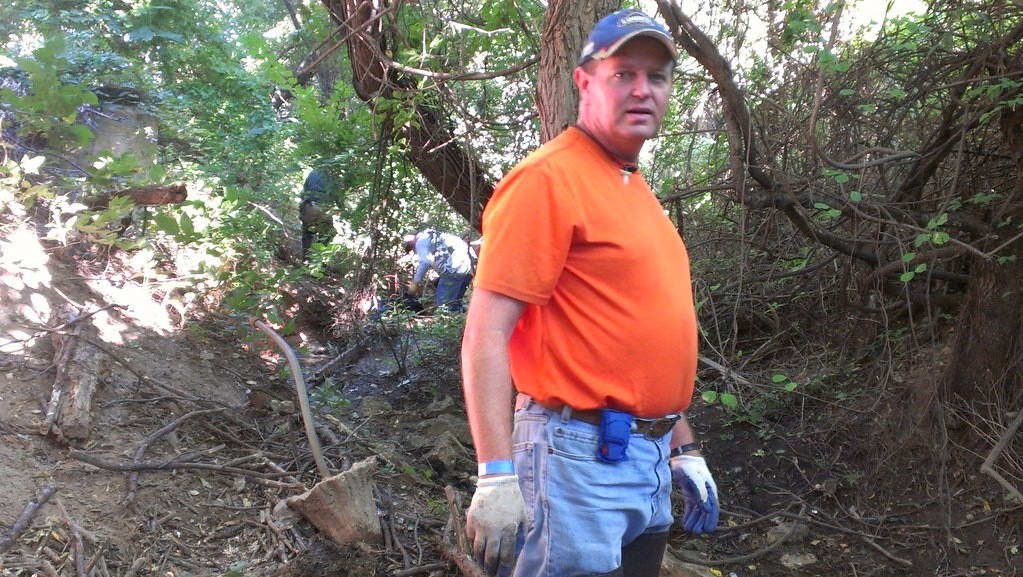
[466,475,529,576]
[670,454,720,535]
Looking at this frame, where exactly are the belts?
[531,399,682,440]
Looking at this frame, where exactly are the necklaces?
[573,124,639,173]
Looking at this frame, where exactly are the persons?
[403,228,473,313]
[300,165,345,262]
[461,8,719,577]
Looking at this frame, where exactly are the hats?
[577,8,676,64]
[401,235,415,254]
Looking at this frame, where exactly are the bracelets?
[668,442,699,458]
[477,460,516,476]
